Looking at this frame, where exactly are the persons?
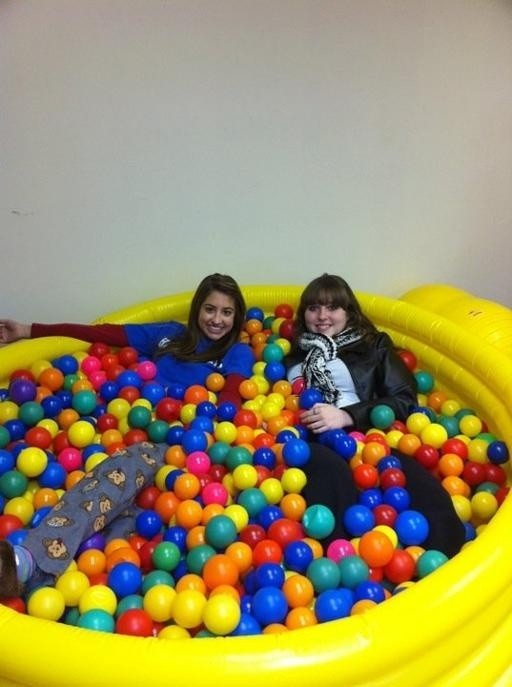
[281,272,465,556]
[0,272,255,596]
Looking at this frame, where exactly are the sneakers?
[1,538,28,599]
